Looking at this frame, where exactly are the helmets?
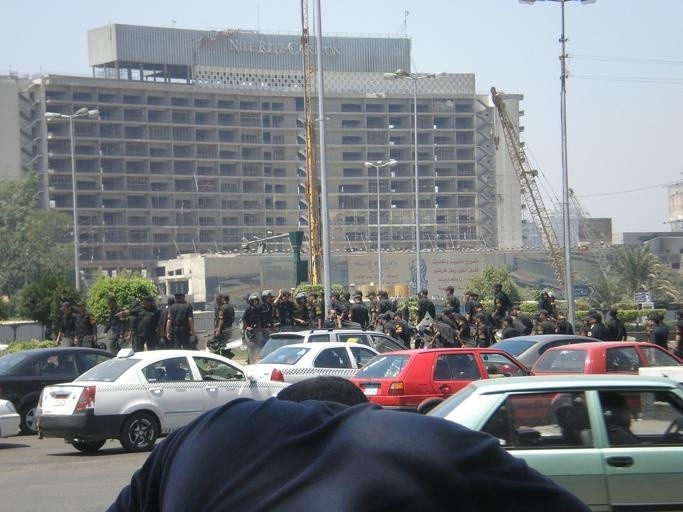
[261,289,276,298]
[248,293,260,305]
[295,293,307,303]
[421,289,429,296]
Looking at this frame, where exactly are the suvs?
[256,329,412,377]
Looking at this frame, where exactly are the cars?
[244,342,399,386]
[473,334,635,376]
[424,376,683,512]
[349,347,582,426]
[1,346,116,434]
[530,341,683,416]
[36,348,293,452]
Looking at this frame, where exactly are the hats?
[59,302,71,311]
[646,316,660,324]
[377,290,389,299]
[445,286,454,292]
[175,293,185,299]
[72,302,86,309]
[223,296,231,302]
[474,313,485,319]
[471,303,483,308]
[500,315,514,323]
[536,309,549,317]
[309,291,318,298]
[242,294,250,300]
[365,291,376,298]
[329,292,340,297]
[492,283,502,289]
[142,296,153,302]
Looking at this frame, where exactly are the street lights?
[363,160,400,286]
[383,69,447,302]
[517,0,599,335]
[45,107,100,290]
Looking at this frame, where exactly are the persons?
[158,297,174,349]
[471,302,494,328]
[71,302,98,348]
[365,290,378,330]
[115,295,161,352]
[578,317,591,338]
[674,310,682,355]
[127,298,144,351]
[324,309,341,328]
[555,311,574,335]
[242,295,249,303]
[375,314,398,339]
[500,317,520,341]
[329,292,348,320]
[475,326,490,349]
[646,316,669,351]
[656,315,664,325]
[402,302,409,322]
[274,289,297,331]
[103,297,125,355]
[319,291,325,316]
[353,290,366,305]
[214,294,224,336]
[512,306,534,335]
[440,310,456,329]
[291,293,310,329]
[415,289,436,348]
[537,309,556,334]
[55,302,77,348]
[306,292,322,329]
[216,294,235,360]
[465,293,482,315]
[444,285,460,313]
[448,307,470,341]
[492,283,509,318]
[539,291,557,316]
[348,295,369,332]
[259,290,274,345]
[589,313,607,341]
[394,312,411,350]
[160,361,178,381]
[427,314,466,349]
[375,290,393,314]
[465,291,472,300]
[603,394,637,445]
[605,308,627,341]
[343,293,353,309]
[242,295,260,364]
[473,313,497,349]
[166,294,194,349]
[106,376,592,511]
